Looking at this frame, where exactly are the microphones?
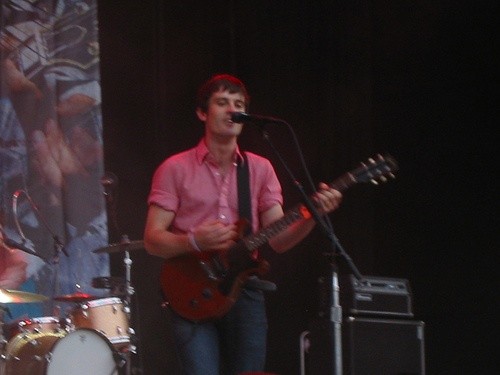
[231,112,284,124]
[53,235,70,257]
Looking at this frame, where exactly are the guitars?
[157,148,395,320]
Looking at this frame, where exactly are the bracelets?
[186,230,202,252]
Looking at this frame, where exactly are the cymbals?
[55,294,95,301]
[92,240,143,253]
[5,236,45,259]
[0,289,50,304]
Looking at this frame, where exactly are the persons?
[144,73,343,375]
[0,0,109,249]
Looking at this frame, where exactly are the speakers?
[306,313,426,375]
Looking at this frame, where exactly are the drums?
[66,297,135,348]
[9,314,68,337]
[0,324,122,374]
